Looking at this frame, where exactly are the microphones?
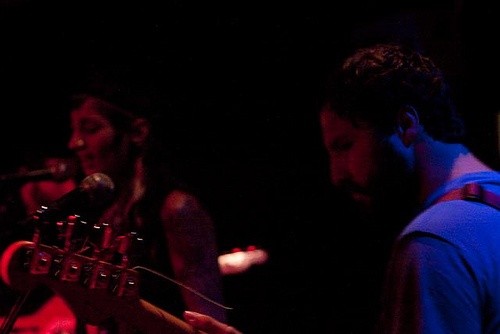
[34,172,115,219]
[0,162,66,183]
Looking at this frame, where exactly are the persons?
[32,87,228,334]
[184,45,500,334]
[20,146,78,220]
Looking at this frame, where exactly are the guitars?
[218,244,267,276]
[0,206,210,334]
